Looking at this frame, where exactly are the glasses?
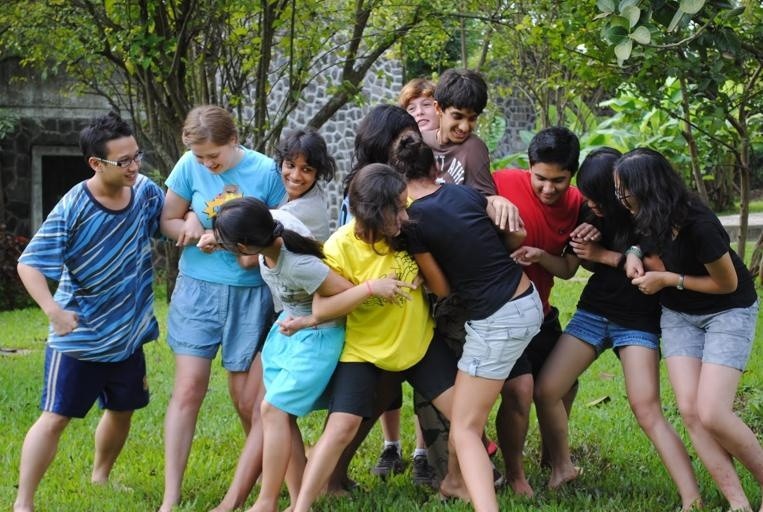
[614,189,639,201]
[95,151,145,168]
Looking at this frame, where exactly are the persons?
[162,68,601,511]
[613,148,763,512]
[11,113,206,512]
[531,148,701,512]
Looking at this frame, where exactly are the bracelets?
[677,274,684,290]
[625,246,643,258]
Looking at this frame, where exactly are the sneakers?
[485,439,498,457]
[411,453,436,485]
[493,468,509,490]
[372,445,404,477]
